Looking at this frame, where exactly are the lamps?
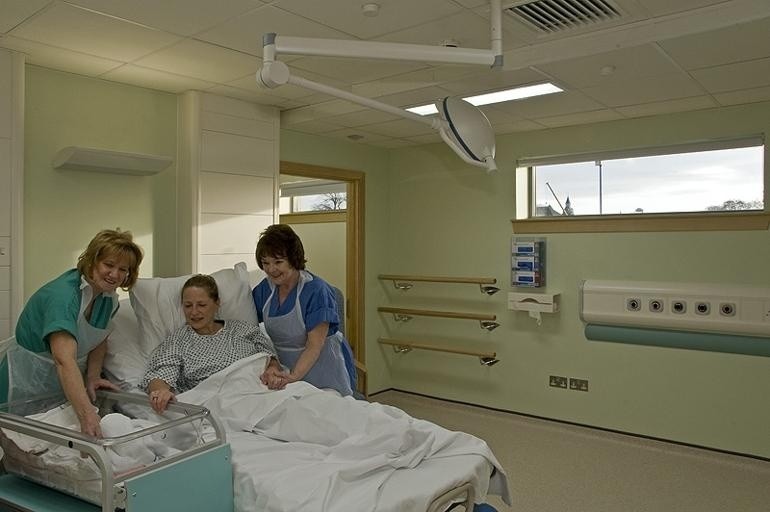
[255,1,506,176]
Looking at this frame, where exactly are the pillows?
[103,262,259,377]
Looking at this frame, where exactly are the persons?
[137,274,286,414]
[0,227,144,458]
[252,223,365,400]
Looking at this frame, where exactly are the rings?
[153,397,158,399]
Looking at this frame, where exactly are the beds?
[102,313,491,510]
